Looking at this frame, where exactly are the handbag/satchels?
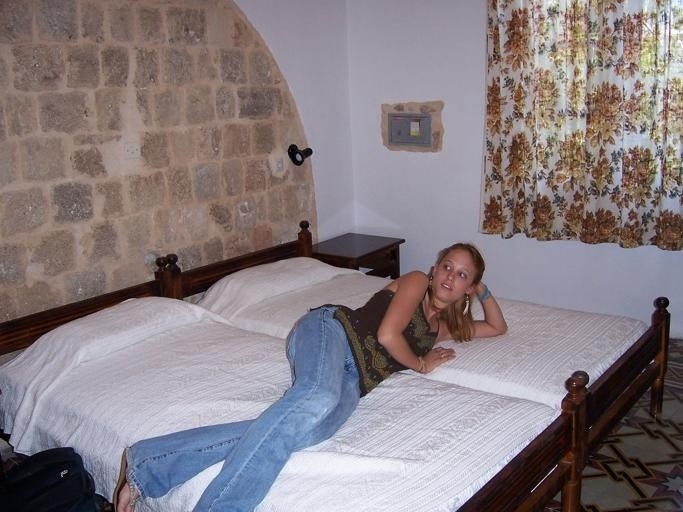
[0,447,104,512]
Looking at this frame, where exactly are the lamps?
[287,143,313,165]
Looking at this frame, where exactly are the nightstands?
[308,228,407,282]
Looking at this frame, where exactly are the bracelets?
[415,356,425,373]
[478,284,491,302]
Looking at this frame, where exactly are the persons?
[113,243,508,512]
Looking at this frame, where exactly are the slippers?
[113,448,141,512]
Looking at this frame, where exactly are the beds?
[1,256,584,511]
[164,221,671,495]
